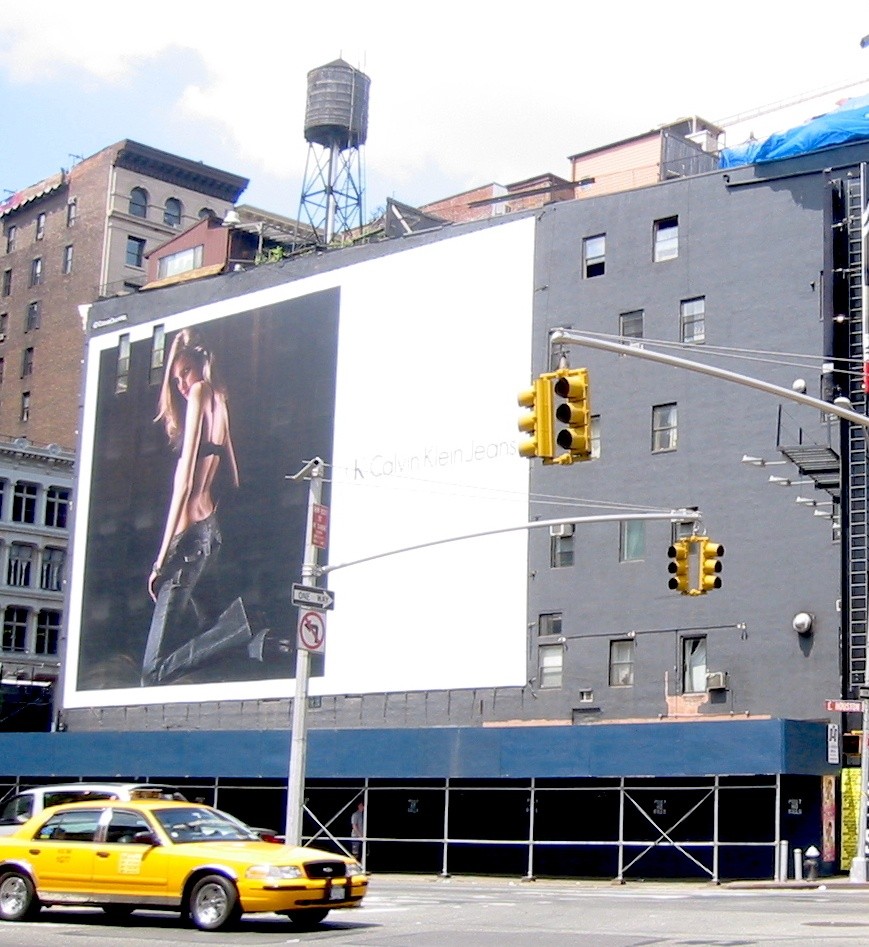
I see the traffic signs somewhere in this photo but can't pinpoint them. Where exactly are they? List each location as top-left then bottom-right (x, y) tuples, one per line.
(292, 582), (335, 611)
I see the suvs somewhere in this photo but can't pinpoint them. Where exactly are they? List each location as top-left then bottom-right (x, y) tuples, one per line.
(0, 782), (201, 833)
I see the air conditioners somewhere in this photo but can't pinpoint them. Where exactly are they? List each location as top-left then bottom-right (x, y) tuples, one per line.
(706, 672), (726, 691)
(550, 524), (573, 538)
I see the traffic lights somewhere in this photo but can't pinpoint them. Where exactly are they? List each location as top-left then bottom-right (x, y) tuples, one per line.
(698, 541), (724, 592)
(553, 372), (591, 456)
(667, 543), (690, 592)
(516, 378), (556, 459)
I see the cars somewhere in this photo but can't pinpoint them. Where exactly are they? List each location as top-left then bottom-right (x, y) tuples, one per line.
(0, 790), (369, 932)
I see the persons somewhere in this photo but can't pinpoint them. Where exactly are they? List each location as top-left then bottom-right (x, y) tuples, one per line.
(351, 802), (372, 875)
(142, 325), (294, 689)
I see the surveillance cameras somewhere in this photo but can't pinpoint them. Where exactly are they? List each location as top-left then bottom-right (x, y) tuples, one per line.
(293, 458), (320, 483)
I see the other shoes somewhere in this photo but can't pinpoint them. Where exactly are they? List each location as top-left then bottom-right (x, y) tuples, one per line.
(263, 633), (295, 664)
